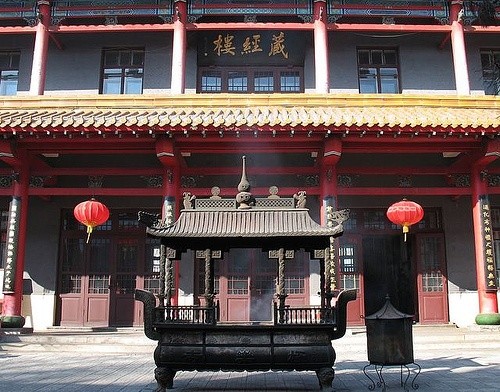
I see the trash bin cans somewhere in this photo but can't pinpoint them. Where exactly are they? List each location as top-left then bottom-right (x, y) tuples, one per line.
(361, 294), (422, 391)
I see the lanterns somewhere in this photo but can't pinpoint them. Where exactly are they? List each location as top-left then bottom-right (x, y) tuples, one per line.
(73, 195), (110, 244)
(386, 196), (424, 241)
